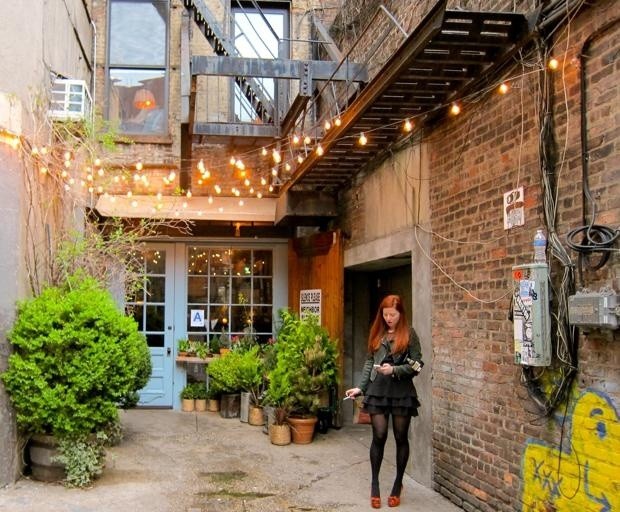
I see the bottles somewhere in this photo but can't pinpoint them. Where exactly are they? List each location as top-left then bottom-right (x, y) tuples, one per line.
(532, 231), (547, 263)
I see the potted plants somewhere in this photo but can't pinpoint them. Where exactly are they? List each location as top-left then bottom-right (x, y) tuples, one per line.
(179, 305), (337, 445)
(4, 269), (152, 482)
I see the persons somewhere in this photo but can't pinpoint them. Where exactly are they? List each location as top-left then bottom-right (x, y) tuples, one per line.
(134, 298), (272, 348)
(345, 294), (425, 509)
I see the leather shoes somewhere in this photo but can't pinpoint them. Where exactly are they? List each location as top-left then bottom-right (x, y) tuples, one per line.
(388, 484), (403, 507)
(371, 496), (381, 508)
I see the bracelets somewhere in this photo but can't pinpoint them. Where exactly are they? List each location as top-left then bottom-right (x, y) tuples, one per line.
(391, 366), (395, 377)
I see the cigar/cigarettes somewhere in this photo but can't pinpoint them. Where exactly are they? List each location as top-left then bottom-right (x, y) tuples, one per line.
(342, 396), (351, 401)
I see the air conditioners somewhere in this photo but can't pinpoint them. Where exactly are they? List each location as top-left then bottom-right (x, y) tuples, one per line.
(47, 76), (94, 139)
